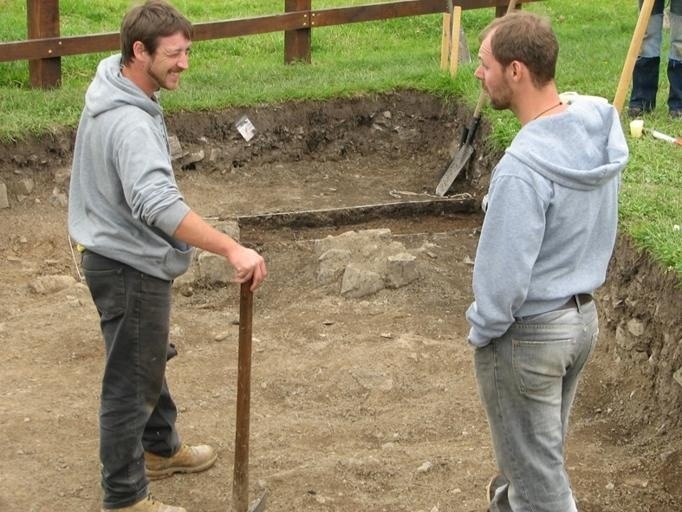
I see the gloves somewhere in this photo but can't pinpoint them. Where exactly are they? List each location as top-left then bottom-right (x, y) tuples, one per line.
(564, 294), (592, 307)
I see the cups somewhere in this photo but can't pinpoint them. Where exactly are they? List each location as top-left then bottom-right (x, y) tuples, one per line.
(630, 119), (643, 138)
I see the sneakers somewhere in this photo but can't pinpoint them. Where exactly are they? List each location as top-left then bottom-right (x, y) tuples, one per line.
(668, 107), (682, 115)
(630, 107), (643, 117)
(487, 474), (507, 504)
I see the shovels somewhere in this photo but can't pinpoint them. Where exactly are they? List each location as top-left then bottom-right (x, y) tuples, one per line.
(435, 0), (517, 197)
(445, 0), (471, 67)
(630, 119), (682, 146)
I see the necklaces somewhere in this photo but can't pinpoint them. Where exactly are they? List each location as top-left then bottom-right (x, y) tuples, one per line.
(532, 101), (563, 120)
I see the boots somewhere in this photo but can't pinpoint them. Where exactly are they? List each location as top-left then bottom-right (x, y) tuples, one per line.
(103, 497), (187, 512)
(144, 444), (217, 481)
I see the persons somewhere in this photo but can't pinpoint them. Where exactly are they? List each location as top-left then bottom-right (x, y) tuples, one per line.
(466, 9), (629, 512)
(628, 0), (682, 117)
(68, 0), (268, 512)
